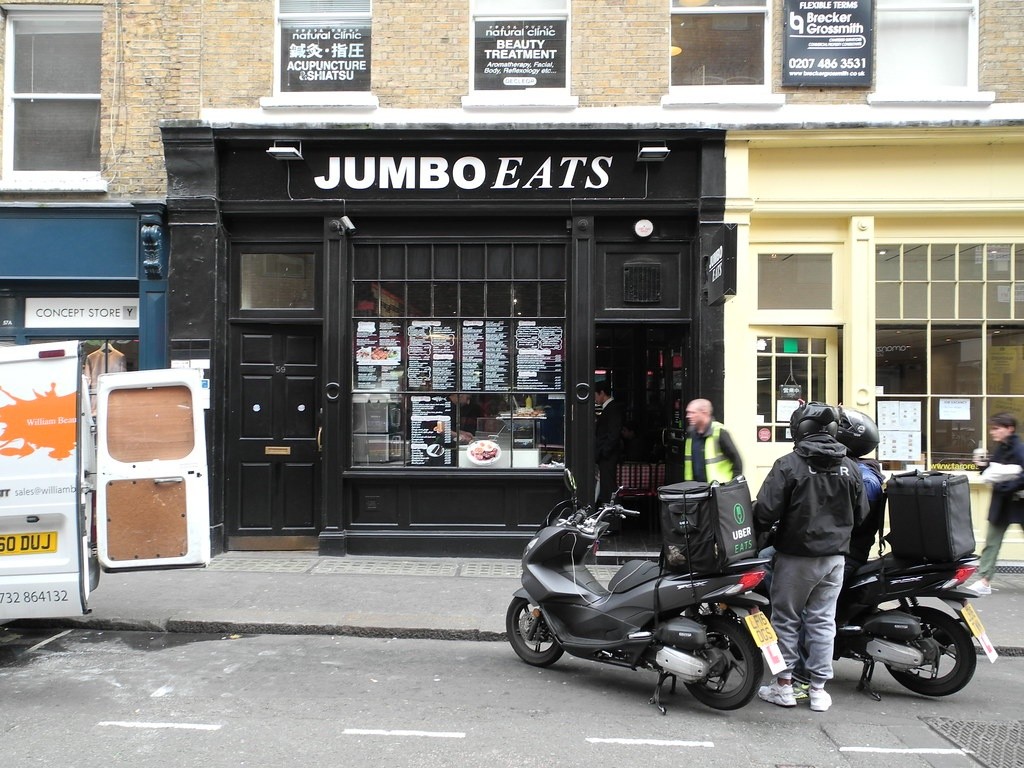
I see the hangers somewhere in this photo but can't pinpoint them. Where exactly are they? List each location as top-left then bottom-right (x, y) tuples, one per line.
(99, 341), (114, 351)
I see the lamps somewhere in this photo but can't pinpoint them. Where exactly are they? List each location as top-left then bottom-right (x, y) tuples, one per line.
(265, 139), (303, 161)
(671, 36), (682, 57)
(636, 140), (671, 162)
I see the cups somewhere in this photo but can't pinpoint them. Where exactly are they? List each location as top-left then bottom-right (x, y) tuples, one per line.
(973, 448), (989, 467)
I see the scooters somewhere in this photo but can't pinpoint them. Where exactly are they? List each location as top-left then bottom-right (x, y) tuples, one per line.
(745, 492), (983, 700)
(504, 455), (772, 715)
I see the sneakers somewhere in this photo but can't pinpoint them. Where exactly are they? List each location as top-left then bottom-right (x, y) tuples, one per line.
(757, 677), (799, 707)
(808, 687), (832, 712)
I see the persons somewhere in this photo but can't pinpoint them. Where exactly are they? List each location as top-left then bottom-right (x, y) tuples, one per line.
(594, 382), (624, 517)
(965, 414), (1024, 595)
(776, 408), (885, 700)
(748, 401), (863, 711)
(621, 420), (648, 462)
(675, 399), (743, 491)
(426, 390), (474, 467)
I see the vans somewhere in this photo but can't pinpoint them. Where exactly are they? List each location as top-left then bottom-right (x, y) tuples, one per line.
(1, 342), (196, 609)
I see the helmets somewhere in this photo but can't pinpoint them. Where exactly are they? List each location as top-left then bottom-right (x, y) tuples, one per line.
(832, 404), (881, 458)
(788, 401), (840, 444)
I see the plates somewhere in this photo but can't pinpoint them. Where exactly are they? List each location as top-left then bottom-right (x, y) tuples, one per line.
(466, 440), (501, 466)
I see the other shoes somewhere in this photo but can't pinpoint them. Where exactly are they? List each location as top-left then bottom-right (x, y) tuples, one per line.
(966, 581), (993, 595)
